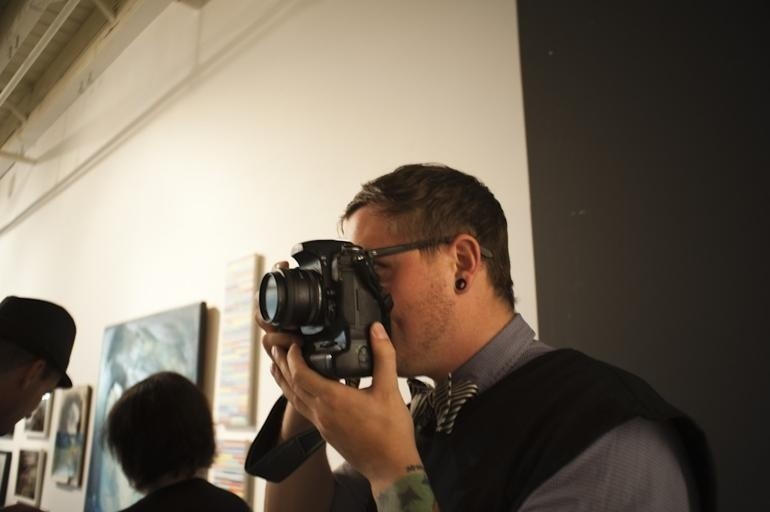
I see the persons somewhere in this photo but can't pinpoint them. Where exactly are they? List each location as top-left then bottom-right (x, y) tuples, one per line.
(0, 296), (76, 437)
(254, 165), (716, 512)
(53, 392), (83, 484)
(106, 371), (252, 512)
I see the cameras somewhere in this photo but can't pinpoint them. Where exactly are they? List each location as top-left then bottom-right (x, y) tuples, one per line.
(258, 239), (393, 379)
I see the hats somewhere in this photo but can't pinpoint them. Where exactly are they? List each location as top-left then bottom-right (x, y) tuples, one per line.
(1, 296), (76, 388)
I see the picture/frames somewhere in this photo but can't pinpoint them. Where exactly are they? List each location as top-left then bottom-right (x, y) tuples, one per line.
(11, 390), (54, 508)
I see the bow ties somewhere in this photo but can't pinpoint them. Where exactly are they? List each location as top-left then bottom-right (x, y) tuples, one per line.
(407, 372), (478, 435)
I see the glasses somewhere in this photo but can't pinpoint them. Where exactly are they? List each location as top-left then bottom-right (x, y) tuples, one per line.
(365, 235), (494, 259)
